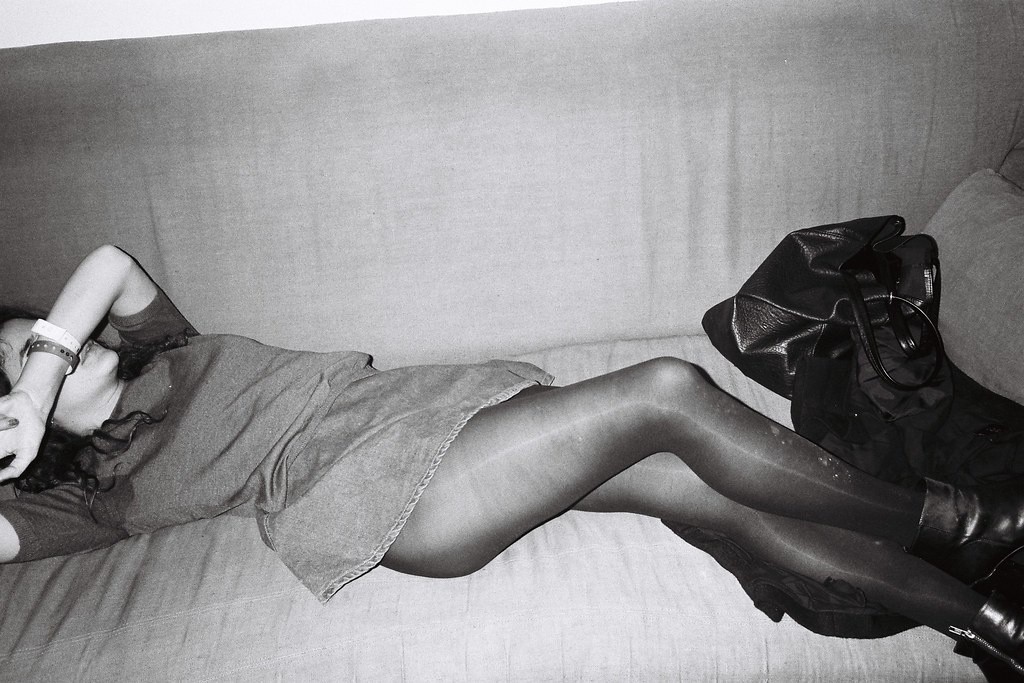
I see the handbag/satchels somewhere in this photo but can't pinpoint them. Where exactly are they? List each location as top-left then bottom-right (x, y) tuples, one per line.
(704, 215), (942, 401)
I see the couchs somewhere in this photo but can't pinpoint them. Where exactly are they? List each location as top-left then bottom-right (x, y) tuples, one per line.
(0, 0), (1024, 683)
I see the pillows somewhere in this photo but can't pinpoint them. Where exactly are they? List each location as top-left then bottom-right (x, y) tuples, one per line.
(921, 167), (1024, 408)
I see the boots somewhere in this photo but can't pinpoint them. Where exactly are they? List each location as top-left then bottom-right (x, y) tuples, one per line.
(952, 588), (1024, 683)
(905, 474), (1023, 594)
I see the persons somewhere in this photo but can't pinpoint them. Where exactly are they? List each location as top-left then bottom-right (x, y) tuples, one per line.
(0, 246), (1024, 683)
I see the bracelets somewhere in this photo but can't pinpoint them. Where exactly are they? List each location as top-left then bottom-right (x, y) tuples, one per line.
(20, 318), (82, 375)
